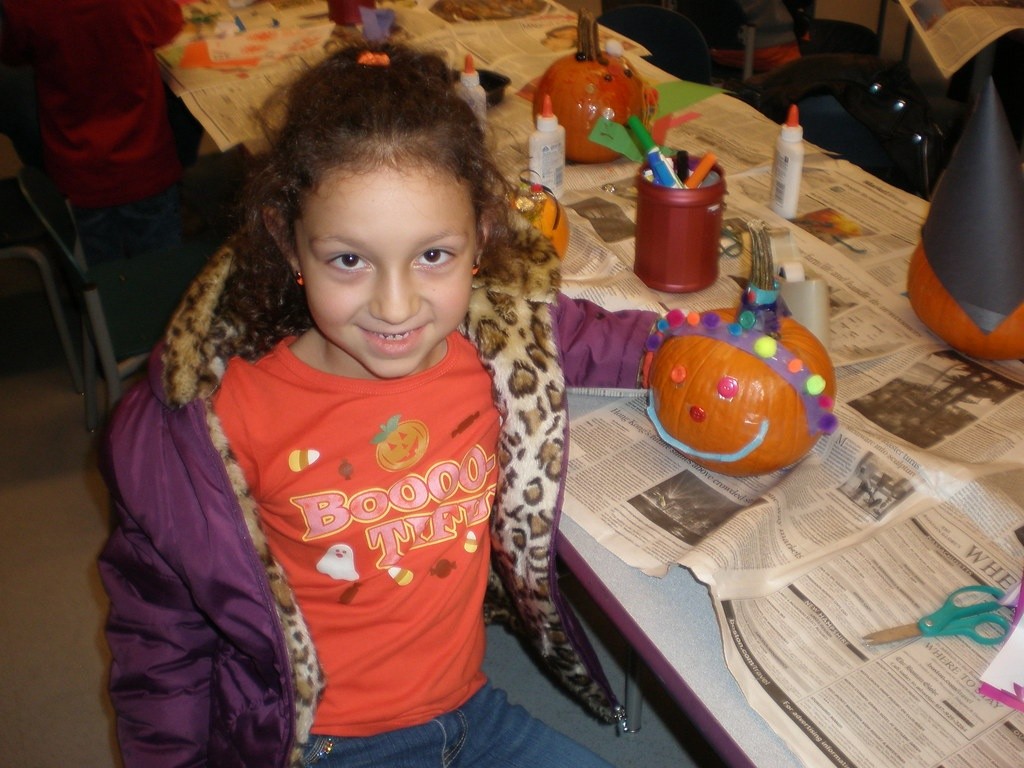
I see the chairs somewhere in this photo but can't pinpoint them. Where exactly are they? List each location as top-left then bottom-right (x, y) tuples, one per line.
(797, 19), (880, 56)
(596, 6), (712, 86)
(17, 161), (218, 432)
(0, 133), (85, 396)
(727, 51), (943, 198)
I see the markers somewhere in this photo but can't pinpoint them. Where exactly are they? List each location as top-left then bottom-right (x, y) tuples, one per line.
(626, 112), (722, 192)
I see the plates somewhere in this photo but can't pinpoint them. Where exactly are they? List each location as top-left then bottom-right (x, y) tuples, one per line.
(453, 68), (512, 108)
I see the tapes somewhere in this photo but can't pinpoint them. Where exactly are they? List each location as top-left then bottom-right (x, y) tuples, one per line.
(776, 261), (806, 281)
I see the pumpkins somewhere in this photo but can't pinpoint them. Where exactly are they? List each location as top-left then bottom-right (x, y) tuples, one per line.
(907, 243), (1024, 361)
(648, 218), (838, 480)
(532, 8), (648, 164)
(507, 188), (570, 262)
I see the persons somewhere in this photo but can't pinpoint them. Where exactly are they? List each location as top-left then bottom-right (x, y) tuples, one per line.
(709, 0), (811, 73)
(0, 0), (203, 378)
(97, 38), (661, 768)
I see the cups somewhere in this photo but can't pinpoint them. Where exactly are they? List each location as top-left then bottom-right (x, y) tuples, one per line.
(328, 0), (374, 25)
(634, 156), (724, 294)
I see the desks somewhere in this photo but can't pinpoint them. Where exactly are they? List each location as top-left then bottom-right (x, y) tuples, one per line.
(144, 0), (1024, 768)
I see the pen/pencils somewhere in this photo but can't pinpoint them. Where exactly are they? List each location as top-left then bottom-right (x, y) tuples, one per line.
(235, 15), (246, 31)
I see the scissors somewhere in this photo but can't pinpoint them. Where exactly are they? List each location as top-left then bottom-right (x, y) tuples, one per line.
(861, 585), (1011, 648)
